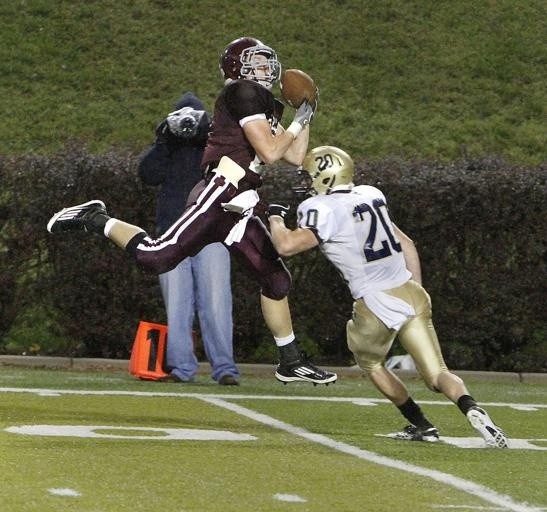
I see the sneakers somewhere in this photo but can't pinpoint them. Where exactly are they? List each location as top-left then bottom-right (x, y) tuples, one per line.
(276, 351), (338, 386)
(385, 425), (439, 441)
(46, 200), (107, 238)
(467, 405), (508, 448)
(159, 375), (179, 381)
(220, 374), (238, 385)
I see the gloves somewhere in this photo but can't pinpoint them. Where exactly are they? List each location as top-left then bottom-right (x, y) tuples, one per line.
(293, 88), (319, 128)
(265, 201), (290, 220)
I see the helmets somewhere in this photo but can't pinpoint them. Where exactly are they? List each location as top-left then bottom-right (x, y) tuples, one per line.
(290, 145), (354, 203)
(219, 37), (275, 90)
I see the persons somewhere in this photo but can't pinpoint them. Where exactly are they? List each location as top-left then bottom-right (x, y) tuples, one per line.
(138, 90), (240, 384)
(264, 146), (510, 449)
(47, 36), (338, 384)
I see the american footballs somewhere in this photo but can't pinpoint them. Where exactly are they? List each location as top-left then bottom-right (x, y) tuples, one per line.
(280, 69), (317, 108)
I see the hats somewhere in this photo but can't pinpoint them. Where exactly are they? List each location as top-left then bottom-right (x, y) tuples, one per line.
(176, 93), (208, 128)
